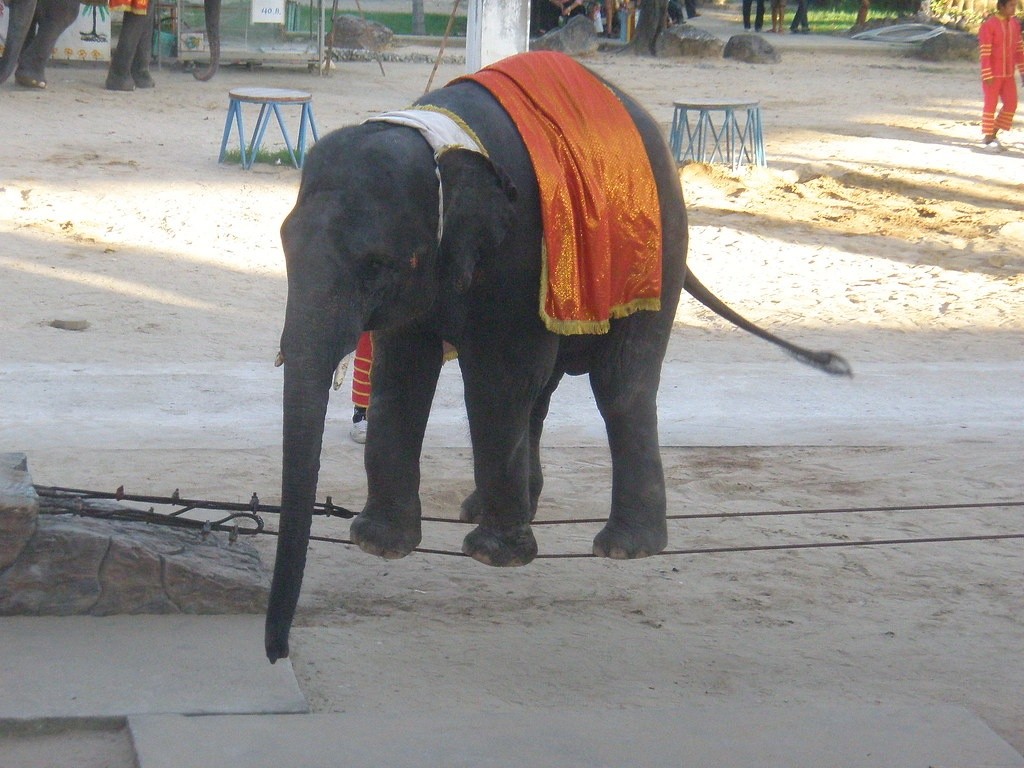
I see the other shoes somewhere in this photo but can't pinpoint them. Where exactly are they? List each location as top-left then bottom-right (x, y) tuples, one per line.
(802, 27), (810, 33)
(790, 27), (802, 33)
(745, 28), (751, 33)
(985, 137), (1007, 154)
(665, 11), (701, 27)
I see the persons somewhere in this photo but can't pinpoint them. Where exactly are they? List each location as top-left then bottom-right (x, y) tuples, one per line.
(536, 0), (613, 37)
(767, 0), (787, 34)
(668, 0), (686, 25)
(976, 0), (1024, 150)
(743, 0), (765, 33)
(790, 0), (811, 34)
(686, 0), (701, 18)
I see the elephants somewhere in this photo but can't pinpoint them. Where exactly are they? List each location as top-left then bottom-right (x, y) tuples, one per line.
(264, 49), (855, 666)
(0, 0), (222, 92)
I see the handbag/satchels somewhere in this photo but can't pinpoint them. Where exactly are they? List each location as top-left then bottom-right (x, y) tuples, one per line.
(558, 14), (567, 27)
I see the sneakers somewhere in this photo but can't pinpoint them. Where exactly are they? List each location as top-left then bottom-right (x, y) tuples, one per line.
(350, 416), (368, 443)
